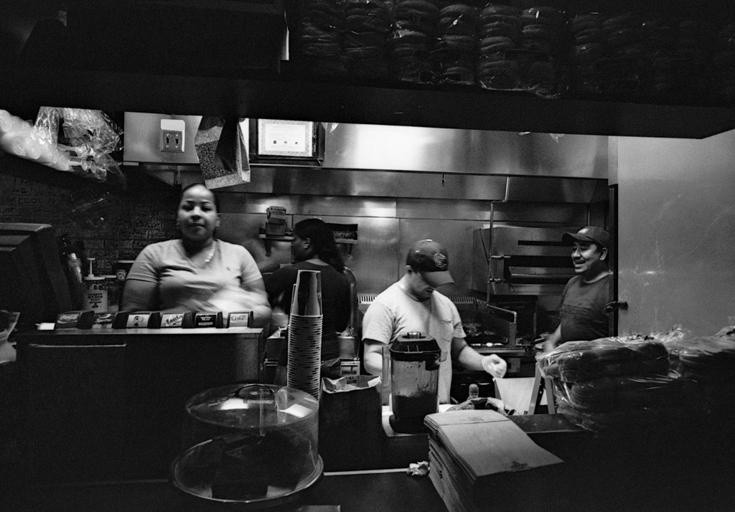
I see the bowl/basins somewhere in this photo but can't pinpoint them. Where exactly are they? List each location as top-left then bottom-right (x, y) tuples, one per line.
(169, 438), (324, 504)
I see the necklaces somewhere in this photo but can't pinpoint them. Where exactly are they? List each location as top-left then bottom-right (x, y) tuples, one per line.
(201, 241), (218, 268)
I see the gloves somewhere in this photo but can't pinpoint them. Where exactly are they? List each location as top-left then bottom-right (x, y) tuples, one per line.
(481, 354), (507, 384)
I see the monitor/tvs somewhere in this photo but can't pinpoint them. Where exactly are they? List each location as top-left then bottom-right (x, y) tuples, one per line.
(0, 222), (72, 327)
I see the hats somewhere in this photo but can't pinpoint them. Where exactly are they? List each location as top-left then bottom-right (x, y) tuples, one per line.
(561, 225), (611, 251)
(407, 239), (456, 288)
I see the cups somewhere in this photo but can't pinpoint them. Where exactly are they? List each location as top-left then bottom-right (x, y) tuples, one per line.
(288, 268), (324, 404)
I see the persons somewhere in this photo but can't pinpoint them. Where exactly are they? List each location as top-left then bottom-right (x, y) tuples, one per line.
(265, 217), (351, 386)
(121, 181), (271, 319)
(542, 224), (615, 353)
(361, 238), (509, 404)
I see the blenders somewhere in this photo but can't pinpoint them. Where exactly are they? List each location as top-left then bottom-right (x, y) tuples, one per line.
(386, 332), (444, 435)
